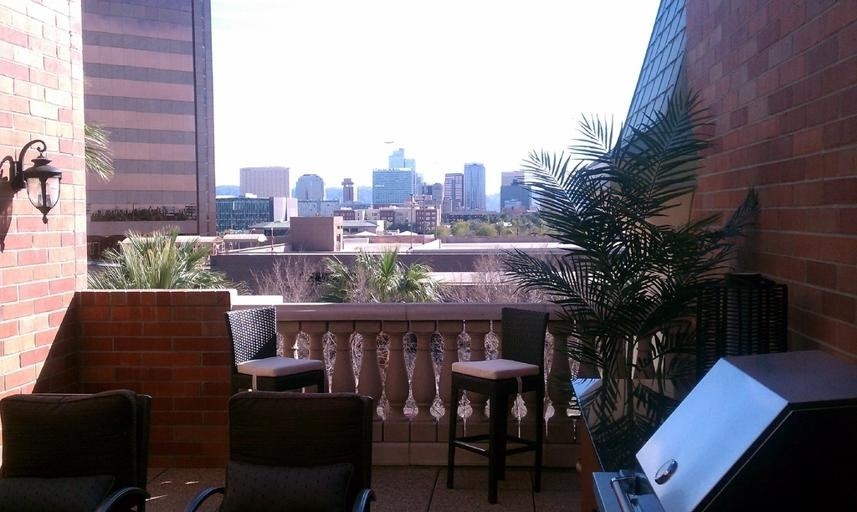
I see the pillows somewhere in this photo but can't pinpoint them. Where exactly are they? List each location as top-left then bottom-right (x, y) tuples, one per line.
(0, 389), (137, 495)
(229, 392), (365, 468)
(0, 475), (117, 512)
(219, 461), (353, 511)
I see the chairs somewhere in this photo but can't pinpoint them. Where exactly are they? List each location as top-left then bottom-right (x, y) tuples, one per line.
(446, 307), (549, 505)
(0, 389), (153, 512)
(223, 305), (325, 394)
(185, 391), (377, 512)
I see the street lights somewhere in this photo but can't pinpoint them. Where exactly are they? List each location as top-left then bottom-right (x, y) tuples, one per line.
(422, 193), (425, 245)
(408, 193), (413, 248)
(433, 199), (437, 240)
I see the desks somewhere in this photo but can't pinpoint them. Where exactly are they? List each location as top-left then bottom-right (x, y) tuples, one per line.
(571, 376), (700, 512)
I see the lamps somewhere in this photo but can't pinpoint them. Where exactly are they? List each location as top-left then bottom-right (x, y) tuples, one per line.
(9, 139), (63, 224)
